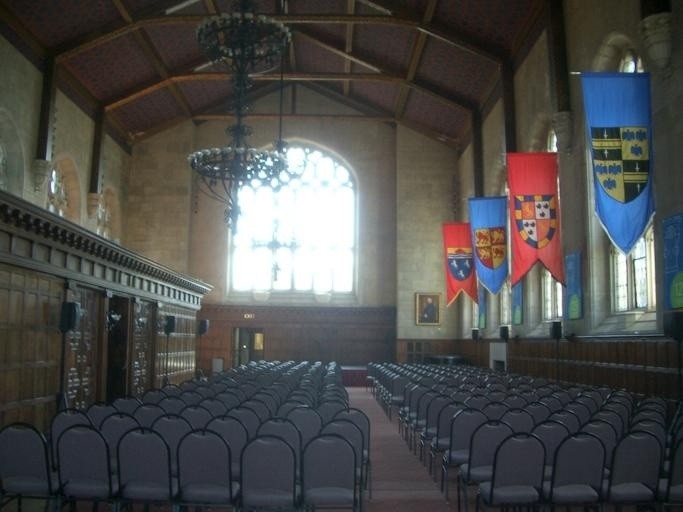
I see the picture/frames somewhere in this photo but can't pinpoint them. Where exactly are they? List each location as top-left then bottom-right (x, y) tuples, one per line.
(415, 292), (442, 326)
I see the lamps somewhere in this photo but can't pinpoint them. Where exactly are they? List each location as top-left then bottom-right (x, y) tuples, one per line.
(178, 6), (299, 235)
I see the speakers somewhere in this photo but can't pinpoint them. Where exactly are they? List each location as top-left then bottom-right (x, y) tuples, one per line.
(663, 310), (683, 340)
(472, 330), (479, 341)
(199, 319), (209, 335)
(63, 302), (81, 330)
(499, 325), (509, 341)
(552, 321), (561, 338)
(164, 316), (176, 334)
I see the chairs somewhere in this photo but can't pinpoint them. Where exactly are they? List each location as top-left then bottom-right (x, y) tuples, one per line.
(367, 361), (683, 511)
(1, 360), (370, 511)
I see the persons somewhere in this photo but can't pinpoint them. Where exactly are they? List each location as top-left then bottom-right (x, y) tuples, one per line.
(422, 296), (435, 323)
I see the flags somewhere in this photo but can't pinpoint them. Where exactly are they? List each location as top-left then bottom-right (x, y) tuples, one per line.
(443, 224), (479, 307)
(511, 153), (566, 289)
(469, 197), (509, 295)
(581, 73), (658, 254)
